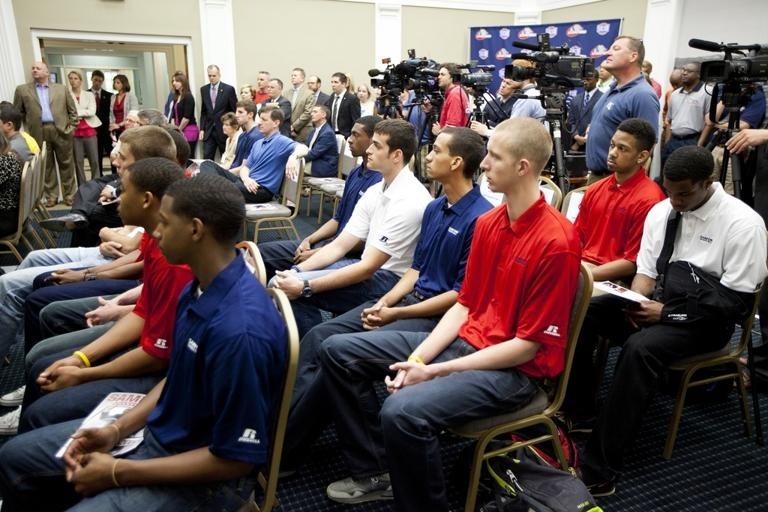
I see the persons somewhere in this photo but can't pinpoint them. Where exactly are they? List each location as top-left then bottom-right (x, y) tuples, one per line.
(398, 59), (547, 199)
(320, 115), (582, 511)
(270, 119), (434, 340)
(199, 65), (239, 161)
(110, 75), (139, 141)
(662, 61), (768, 222)
(1, 102), (190, 434)
(585, 33), (661, 185)
(641, 61), (662, 99)
(572, 119), (665, 282)
(281, 125), (487, 472)
(562, 146), (767, 496)
(222, 68), (377, 204)
(3, 173), (289, 511)
(13, 61), (80, 206)
(88, 69), (117, 175)
(68, 71), (101, 186)
(164, 72), (200, 168)
(19, 158), (194, 437)
(564, 60), (617, 190)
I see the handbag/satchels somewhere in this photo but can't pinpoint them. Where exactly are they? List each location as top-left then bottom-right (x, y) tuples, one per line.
(656, 259), (757, 325)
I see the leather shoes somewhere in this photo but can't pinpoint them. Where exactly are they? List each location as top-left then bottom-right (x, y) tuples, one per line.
(38, 214), (89, 233)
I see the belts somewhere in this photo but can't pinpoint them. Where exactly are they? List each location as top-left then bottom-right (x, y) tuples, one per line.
(42, 121), (54, 126)
(413, 290), (425, 301)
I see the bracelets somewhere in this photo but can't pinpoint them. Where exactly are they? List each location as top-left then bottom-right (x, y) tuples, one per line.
(112, 460), (121, 485)
(73, 351), (90, 366)
(83, 273), (87, 281)
(110, 424), (120, 442)
(291, 267), (299, 272)
(88, 272), (96, 281)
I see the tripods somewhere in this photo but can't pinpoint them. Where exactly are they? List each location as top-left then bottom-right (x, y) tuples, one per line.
(414, 98), (444, 157)
(407, 93), (429, 183)
(704, 105), (763, 448)
(532, 120), (567, 199)
(382, 96), (404, 120)
(434, 109), (492, 198)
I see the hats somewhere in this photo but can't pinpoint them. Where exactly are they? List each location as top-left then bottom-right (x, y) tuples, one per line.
(512, 59), (534, 84)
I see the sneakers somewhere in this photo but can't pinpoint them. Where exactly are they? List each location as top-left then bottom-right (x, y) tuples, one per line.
(553, 410), (592, 434)
(568, 467), (616, 497)
(0, 383), (27, 407)
(0, 407), (21, 434)
(327, 469), (394, 505)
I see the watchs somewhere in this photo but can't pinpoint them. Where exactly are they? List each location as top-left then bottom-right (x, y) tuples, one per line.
(301, 280), (313, 298)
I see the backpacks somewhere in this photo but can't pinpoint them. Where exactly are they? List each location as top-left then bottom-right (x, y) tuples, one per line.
(480, 450), (603, 512)
(485, 408), (578, 471)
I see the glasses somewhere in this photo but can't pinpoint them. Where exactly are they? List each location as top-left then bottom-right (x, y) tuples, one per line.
(637, 39), (642, 52)
(683, 69), (696, 73)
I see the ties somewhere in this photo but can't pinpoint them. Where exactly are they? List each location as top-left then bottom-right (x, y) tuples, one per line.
(212, 87), (216, 109)
(332, 96), (339, 131)
(584, 95), (589, 106)
(95, 92), (100, 112)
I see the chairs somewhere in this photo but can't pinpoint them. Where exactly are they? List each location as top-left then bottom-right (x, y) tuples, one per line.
(439, 258), (594, 510)
(241, 153), (306, 244)
(592, 227), (761, 460)
(299, 134), (415, 224)
(0, 141), (59, 268)
(225, 241), (300, 508)
(477, 169), (587, 225)
